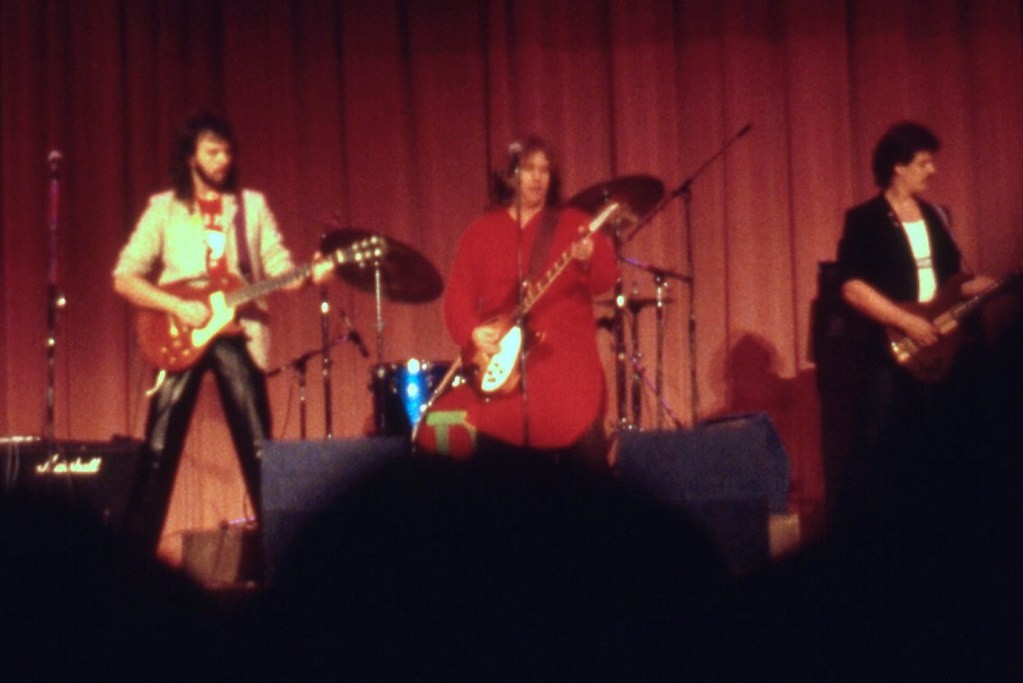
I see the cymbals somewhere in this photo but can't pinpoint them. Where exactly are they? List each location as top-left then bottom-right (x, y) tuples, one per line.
(597, 293), (676, 306)
(321, 227), (445, 304)
(568, 174), (665, 231)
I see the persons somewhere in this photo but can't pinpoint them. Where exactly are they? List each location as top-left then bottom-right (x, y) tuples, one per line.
(109, 109), (335, 593)
(829, 121), (1001, 510)
(446, 135), (656, 604)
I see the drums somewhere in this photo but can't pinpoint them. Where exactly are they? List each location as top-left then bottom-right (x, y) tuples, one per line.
(367, 357), (455, 435)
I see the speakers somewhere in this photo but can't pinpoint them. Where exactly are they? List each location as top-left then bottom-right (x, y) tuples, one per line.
(610, 414), (790, 515)
(0, 438), (143, 526)
(259, 438), (406, 566)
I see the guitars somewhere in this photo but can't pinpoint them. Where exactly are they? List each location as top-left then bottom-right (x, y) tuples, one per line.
(459, 195), (631, 403)
(884, 268), (1023, 384)
(134, 234), (389, 373)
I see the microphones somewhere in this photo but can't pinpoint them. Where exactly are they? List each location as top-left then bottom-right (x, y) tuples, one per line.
(341, 312), (370, 358)
(47, 150), (62, 173)
(508, 142), (523, 173)
